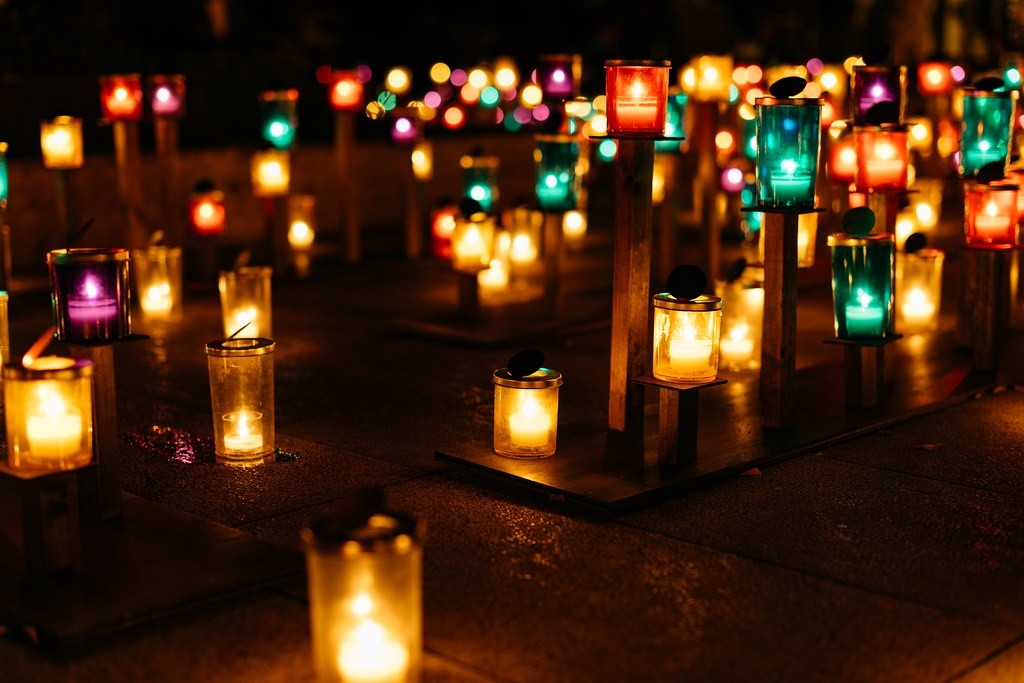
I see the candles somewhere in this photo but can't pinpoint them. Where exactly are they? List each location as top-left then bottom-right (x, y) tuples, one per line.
(508, 399), (552, 446)
(335, 621), (410, 681)
(25, 392), (82, 458)
(720, 322), (753, 363)
(225, 408), (263, 451)
(671, 328), (712, 372)
(1, 57), (1021, 335)
